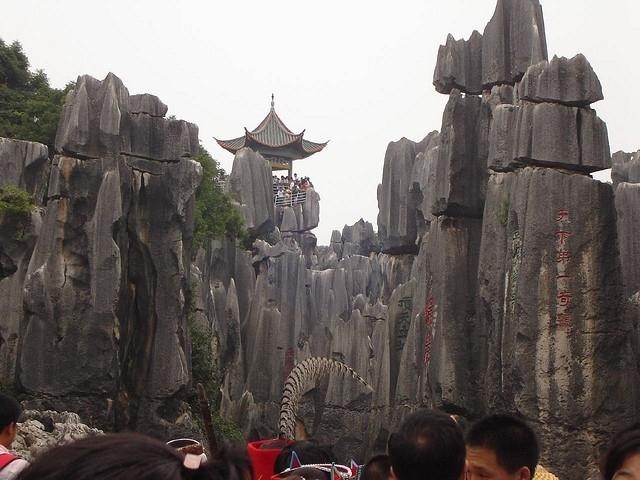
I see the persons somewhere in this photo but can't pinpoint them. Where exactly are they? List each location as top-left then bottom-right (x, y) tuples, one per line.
(273, 173), (313, 208)
